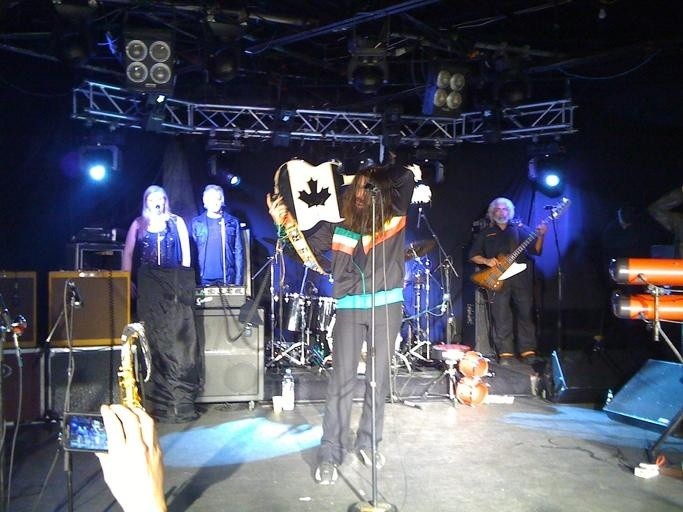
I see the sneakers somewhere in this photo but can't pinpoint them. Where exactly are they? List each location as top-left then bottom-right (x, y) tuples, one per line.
(313, 461), (338, 486)
(360, 446), (385, 469)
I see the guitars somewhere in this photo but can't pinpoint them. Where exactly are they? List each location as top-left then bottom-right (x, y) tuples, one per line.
(469, 197), (570, 294)
(272, 155), (423, 238)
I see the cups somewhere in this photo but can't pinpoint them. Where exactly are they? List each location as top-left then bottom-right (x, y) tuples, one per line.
(272, 395), (284, 413)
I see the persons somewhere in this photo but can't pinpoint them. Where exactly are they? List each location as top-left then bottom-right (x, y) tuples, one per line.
(188, 183), (245, 288)
(467, 196), (546, 364)
(89, 402), (168, 512)
(74, 419), (102, 448)
(263, 162), (416, 486)
(119, 184), (191, 300)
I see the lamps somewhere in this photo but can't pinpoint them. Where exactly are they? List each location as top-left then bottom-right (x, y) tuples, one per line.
(420, 60), (473, 120)
(343, 20), (392, 97)
(121, 28), (178, 95)
(195, 9), (253, 85)
(210, 148), (242, 187)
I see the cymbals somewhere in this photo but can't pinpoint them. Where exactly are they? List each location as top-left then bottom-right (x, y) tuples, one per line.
(405, 240), (436, 262)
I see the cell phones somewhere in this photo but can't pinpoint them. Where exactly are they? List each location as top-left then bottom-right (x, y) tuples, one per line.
(62, 411), (109, 453)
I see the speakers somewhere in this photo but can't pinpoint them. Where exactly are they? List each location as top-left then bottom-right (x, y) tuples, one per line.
(192, 21), (242, 83)
(422, 61), (472, 118)
(348, 47), (390, 94)
(122, 27), (175, 93)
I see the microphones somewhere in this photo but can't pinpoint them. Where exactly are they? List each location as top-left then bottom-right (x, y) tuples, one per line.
(68, 279), (84, 311)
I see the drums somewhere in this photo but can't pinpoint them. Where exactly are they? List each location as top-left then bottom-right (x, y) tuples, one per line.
(327, 311), (402, 375)
(276, 293), (338, 332)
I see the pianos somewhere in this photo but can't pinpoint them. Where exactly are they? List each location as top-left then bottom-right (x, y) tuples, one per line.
(67, 226), (127, 245)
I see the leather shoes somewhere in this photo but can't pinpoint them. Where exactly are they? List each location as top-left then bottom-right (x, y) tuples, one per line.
(498, 350), (535, 359)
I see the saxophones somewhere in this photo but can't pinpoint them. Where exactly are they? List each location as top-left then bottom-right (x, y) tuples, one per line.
(118, 321), (147, 410)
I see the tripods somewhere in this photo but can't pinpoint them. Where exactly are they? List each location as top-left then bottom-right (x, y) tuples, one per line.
(388, 269), (464, 410)
(265, 264), (331, 379)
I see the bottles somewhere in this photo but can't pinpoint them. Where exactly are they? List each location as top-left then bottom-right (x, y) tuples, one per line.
(282, 369), (295, 412)
